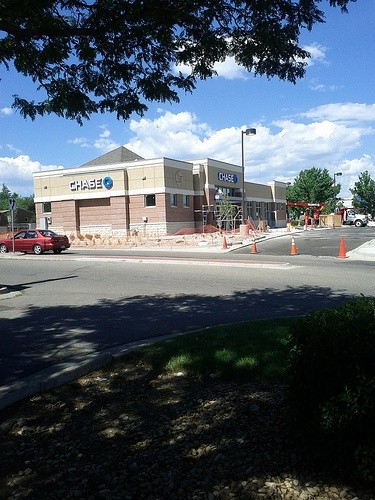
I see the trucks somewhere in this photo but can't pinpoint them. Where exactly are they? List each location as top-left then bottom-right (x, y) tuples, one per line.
(337, 207), (368, 227)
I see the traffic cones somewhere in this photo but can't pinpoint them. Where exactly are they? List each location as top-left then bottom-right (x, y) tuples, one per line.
(222, 237), (228, 249)
(337, 235), (348, 258)
(251, 236), (258, 254)
(290, 237), (298, 254)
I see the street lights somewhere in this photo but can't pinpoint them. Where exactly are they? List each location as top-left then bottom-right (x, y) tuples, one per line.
(239, 128), (257, 235)
(334, 173), (342, 186)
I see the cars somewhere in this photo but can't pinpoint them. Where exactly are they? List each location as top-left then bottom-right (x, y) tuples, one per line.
(0, 229), (70, 255)
